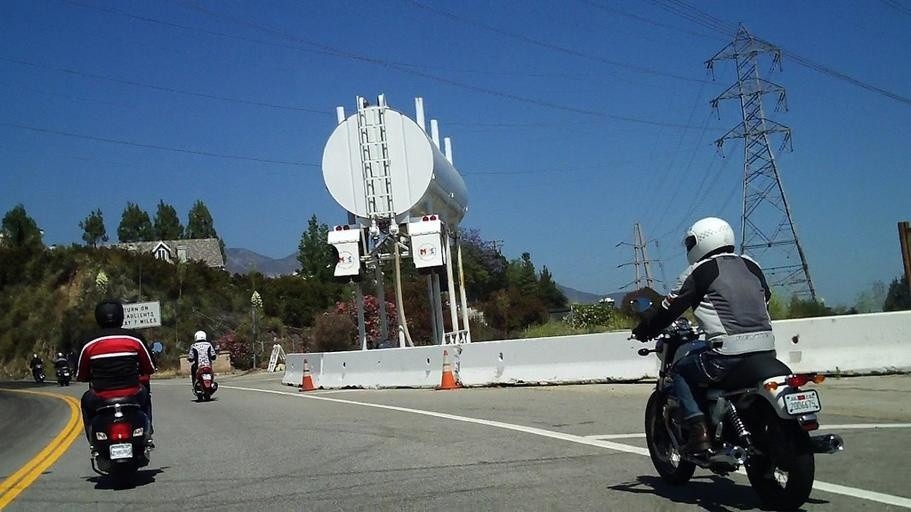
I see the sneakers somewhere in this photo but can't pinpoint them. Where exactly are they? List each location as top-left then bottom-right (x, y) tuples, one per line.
(677, 426), (714, 456)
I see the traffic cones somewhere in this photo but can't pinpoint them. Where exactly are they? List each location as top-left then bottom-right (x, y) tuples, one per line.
(436, 348), (461, 390)
(297, 358), (319, 391)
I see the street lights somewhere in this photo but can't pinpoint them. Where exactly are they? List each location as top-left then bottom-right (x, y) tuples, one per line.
(127, 246), (143, 302)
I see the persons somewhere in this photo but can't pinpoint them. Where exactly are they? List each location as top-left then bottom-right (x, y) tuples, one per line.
(54, 351), (69, 381)
(29, 352), (45, 380)
(75, 299), (158, 456)
(631, 217), (777, 456)
(187, 330), (217, 387)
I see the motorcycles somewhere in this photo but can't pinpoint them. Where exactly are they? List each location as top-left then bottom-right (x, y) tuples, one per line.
(50, 355), (75, 388)
(27, 359), (50, 386)
(81, 340), (167, 476)
(185, 354), (220, 404)
(621, 287), (845, 512)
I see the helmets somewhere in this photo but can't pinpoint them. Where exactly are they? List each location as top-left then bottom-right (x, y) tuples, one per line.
(94, 298), (125, 329)
(193, 329), (208, 343)
(683, 216), (736, 268)
(56, 352), (64, 359)
(33, 353), (38, 359)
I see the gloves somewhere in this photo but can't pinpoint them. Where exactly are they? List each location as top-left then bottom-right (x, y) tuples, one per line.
(631, 325), (654, 343)
